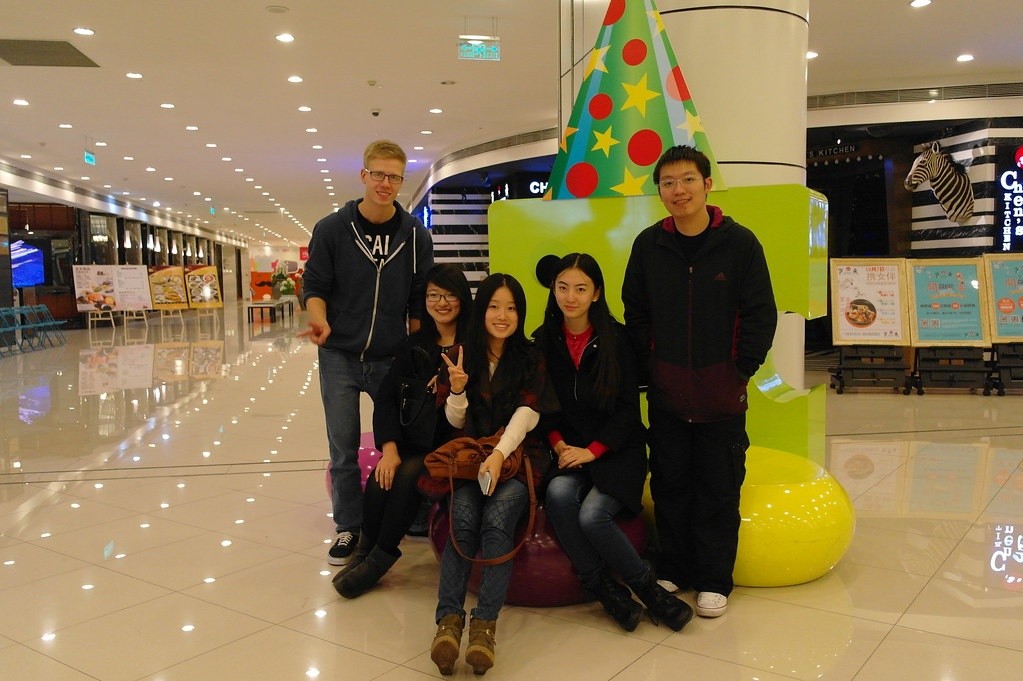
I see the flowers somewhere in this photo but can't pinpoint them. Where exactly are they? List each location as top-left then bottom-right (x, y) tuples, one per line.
(295, 268), (305, 284)
(269, 262), (296, 288)
(280, 278), (296, 295)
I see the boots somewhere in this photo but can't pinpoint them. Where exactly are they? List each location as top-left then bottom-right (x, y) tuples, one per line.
(584, 571), (642, 631)
(465, 619), (497, 675)
(628, 572), (694, 632)
(431, 614), (463, 675)
(332, 527), (403, 599)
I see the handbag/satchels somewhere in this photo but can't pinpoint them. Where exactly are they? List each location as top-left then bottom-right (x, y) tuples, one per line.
(423, 425), (536, 565)
(394, 345), (438, 450)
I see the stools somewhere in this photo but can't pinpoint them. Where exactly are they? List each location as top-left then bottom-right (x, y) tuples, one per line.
(325, 433), (857, 606)
(247, 298), (294, 324)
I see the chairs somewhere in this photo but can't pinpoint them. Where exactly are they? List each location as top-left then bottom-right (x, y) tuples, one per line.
(0, 304), (68, 358)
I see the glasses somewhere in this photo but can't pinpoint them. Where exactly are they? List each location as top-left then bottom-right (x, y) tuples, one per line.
(365, 169), (404, 184)
(659, 175), (703, 189)
(426, 293), (459, 302)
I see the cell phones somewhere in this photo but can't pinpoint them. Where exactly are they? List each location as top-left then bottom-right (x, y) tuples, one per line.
(478, 462), (491, 495)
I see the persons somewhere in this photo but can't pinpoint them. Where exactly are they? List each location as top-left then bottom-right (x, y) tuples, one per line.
(295, 138), (434, 566)
(330, 265), (473, 599)
(539, 253), (695, 633)
(619, 146), (778, 619)
(429, 272), (543, 675)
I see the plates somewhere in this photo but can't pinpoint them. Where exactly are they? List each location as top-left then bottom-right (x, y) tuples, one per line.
(845, 299), (877, 328)
(188, 274), (218, 302)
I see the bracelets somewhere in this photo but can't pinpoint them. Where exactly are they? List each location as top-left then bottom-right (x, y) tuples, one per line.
(450, 389), (466, 395)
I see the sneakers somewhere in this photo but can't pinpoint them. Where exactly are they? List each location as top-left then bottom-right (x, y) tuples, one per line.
(656, 580), (678, 594)
(696, 592), (728, 617)
(328, 531), (360, 565)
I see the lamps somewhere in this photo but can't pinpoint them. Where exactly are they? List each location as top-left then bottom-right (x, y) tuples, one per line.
(92, 218), (204, 259)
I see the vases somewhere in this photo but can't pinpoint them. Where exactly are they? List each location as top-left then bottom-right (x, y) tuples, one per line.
(298, 285), (312, 311)
(271, 279), (292, 311)
(283, 289), (295, 296)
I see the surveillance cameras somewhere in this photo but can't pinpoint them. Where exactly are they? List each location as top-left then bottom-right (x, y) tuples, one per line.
(371, 109), (380, 117)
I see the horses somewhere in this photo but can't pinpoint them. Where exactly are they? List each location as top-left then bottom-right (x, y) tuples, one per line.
(905, 142), (974, 223)
